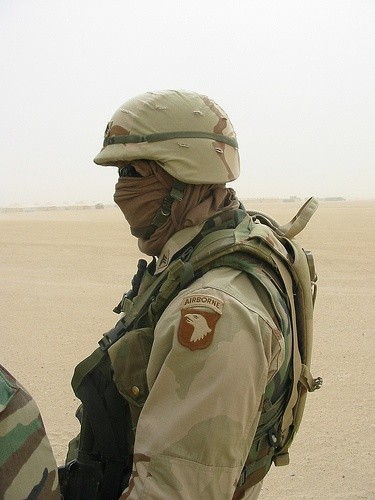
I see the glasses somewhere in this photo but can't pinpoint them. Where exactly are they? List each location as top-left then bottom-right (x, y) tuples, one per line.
(117, 163), (148, 180)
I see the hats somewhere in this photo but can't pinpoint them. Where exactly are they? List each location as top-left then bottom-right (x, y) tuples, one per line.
(92, 91), (239, 184)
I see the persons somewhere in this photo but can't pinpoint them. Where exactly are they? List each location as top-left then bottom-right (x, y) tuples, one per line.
(65, 87), (317, 499)
